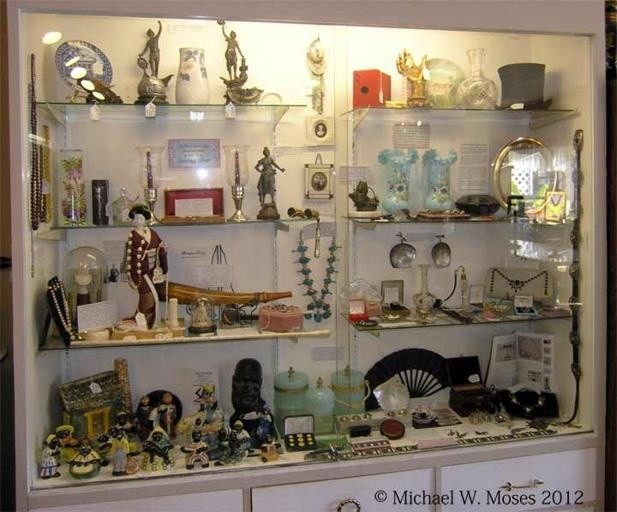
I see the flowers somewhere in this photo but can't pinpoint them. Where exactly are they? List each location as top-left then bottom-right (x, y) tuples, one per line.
(59, 156), (87, 226)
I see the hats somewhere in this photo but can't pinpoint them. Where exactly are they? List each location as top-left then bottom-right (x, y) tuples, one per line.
(494, 63), (552, 110)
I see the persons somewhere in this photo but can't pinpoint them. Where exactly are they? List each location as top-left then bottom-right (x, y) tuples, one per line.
(124, 204), (168, 330)
(254, 148), (285, 205)
(138, 21), (161, 78)
(221, 22), (245, 81)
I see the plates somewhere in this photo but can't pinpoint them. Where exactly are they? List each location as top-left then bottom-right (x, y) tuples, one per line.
(417, 58), (466, 108)
(54, 39), (113, 93)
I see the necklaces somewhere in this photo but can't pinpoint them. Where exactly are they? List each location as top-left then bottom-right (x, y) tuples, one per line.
(30, 54), (39, 230)
(488, 266), (548, 296)
(43, 126), (51, 223)
(509, 392), (546, 412)
(291, 229), (341, 323)
(49, 280), (75, 341)
(39, 145), (46, 222)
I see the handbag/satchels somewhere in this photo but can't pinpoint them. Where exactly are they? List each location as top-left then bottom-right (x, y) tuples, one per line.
(483, 267), (553, 303)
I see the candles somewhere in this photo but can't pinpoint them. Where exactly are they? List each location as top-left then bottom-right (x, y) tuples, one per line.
(146, 151), (153, 187)
(235, 148), (240, 186)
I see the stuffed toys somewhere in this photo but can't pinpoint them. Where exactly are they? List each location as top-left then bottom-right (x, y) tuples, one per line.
(217, 429), (248, 465)
(206, 425), (232, 460)
(192, 300), (212, 324)
(232, 419), (250, 451)
(105, 429), (129, 476)
(180, 430), (208, 469)
(228, 358), (281, 452)
(39, 433), (60, 479)
(157, 391), (177, 435)
(177, 383), (224, 433)
(141, 426), (175, 473)
(138, 395), (154, 439)
(55, 425), (79, 465)
(69, 437), (102, 479)
(108, 410), (139, 456)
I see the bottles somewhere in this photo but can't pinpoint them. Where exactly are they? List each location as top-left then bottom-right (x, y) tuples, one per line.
(91, 178), (110, 225)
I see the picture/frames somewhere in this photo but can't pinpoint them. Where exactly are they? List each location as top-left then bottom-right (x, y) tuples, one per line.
(305, 163), (334, 199)
(306, 114), (334, 145)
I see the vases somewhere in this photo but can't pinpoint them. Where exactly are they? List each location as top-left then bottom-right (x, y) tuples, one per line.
(379, 149), (418, 212)
(412, 265), (436, 312)
(423, 149), (457, 211)
(457, 48), (498, 109)
(174, 44), (209, 106)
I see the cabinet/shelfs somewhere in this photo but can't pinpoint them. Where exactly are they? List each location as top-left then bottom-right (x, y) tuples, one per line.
(26, 486), (245, 512)
(8, 0), (606, 488)
(251, 467), (436, 512)
(439, 446), (598, 512)
